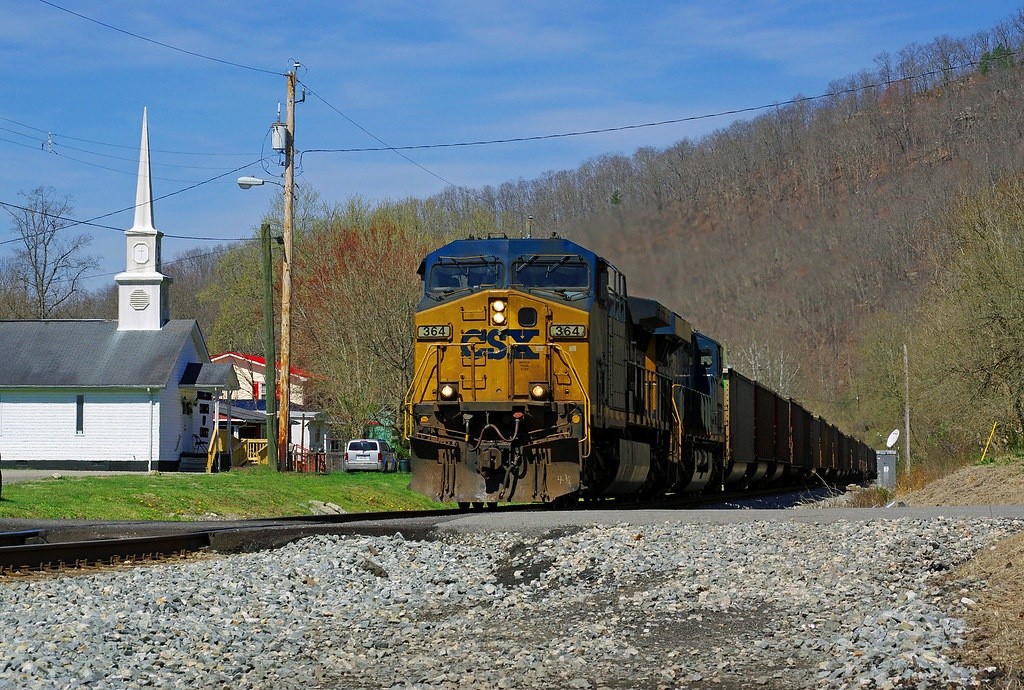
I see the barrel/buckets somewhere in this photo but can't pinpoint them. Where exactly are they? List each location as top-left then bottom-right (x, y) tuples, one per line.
(399, 460), (410, 473)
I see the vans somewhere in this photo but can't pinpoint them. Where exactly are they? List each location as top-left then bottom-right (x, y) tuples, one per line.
(343, 439), (399, 473)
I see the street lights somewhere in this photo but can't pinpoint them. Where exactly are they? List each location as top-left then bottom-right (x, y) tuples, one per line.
(237, 176), (294, 473)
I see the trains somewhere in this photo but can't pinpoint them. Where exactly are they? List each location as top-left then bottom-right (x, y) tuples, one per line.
(402, 235), (878, 510)
